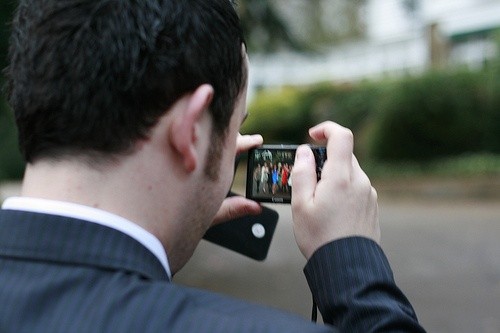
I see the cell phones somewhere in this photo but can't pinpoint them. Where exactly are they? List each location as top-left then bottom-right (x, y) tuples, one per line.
(202, 191), (279, 262)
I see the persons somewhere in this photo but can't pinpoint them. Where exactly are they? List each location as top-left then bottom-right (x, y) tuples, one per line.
(0, 1), (426, 332)
(253, 156), (294, 197)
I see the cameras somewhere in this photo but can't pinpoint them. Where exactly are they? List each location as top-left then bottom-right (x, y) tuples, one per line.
(245, 145), (327, 205)
